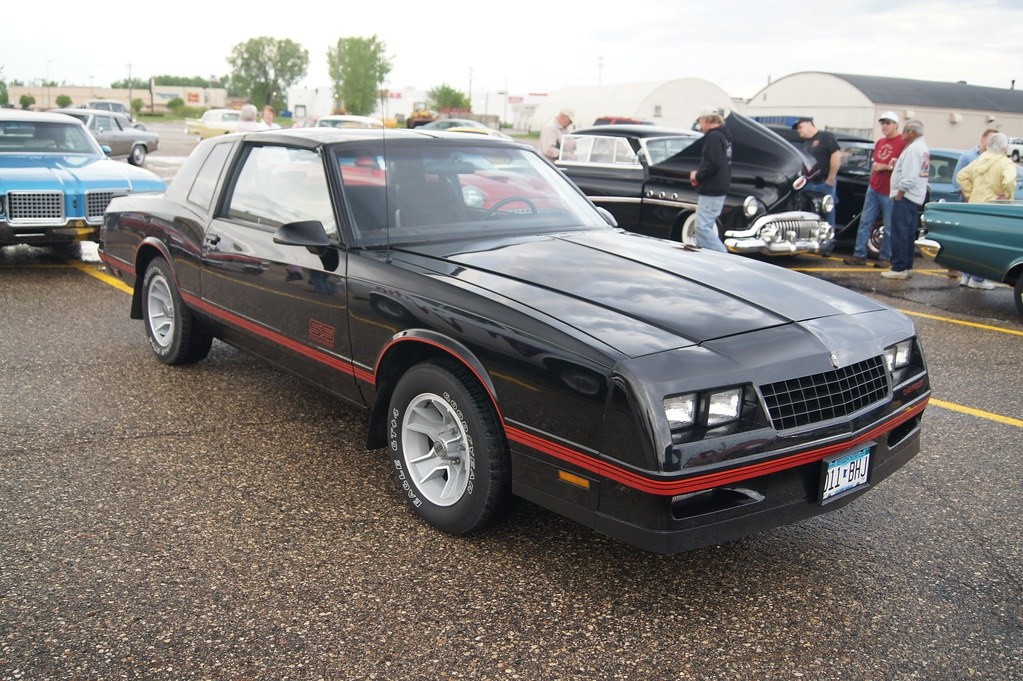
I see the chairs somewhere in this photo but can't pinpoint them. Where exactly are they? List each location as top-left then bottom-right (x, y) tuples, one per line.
(935, 164), (949, 180)
(928, 164), (936, 180)
(396, 178), (468, 227)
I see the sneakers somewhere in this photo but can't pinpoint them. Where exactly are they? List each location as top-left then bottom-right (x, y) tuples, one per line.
(881, 270), (908, 279)
(960, 276), (969, 285)
(907, 270), (912, 277)
(967, 278), (994, 289)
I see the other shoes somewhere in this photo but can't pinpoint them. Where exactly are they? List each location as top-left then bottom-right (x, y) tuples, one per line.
(844, 257), (866, 265)
(874, 258), (890, 268)
(948, 270), (958, 278)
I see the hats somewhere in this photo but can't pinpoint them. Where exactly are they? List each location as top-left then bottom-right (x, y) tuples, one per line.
(792, 118), (812, 130)
(879, 111), (899, 124)
(562, 105), (581, 124)
(696, 107), (718, 122)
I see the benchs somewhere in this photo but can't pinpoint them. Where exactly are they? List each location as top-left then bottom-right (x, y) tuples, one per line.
(0, 138), (57, 149)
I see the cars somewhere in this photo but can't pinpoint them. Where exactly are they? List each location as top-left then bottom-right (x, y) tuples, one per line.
(84, 98), (132, 122)
(0, 106), (166, 257)
(183, 108), (267, 143)
(289, 110), (1023, 322)
(94, 128), (933, 555)
(32, 107), (160, 168)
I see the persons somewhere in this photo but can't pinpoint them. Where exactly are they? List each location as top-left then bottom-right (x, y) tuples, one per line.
(947, 128), (999, 278)
(259, 105), (281, 131)
(880, 121), (929, 279)
(233, 104), (260, 133)
(792, 118), (841, 257)
(305, 268), (339, 297)
(844, 111), (908, 268)
(691, 104), (734, 255)
(537, 107), (577, 164)
(956, 133), (1017, 289)
